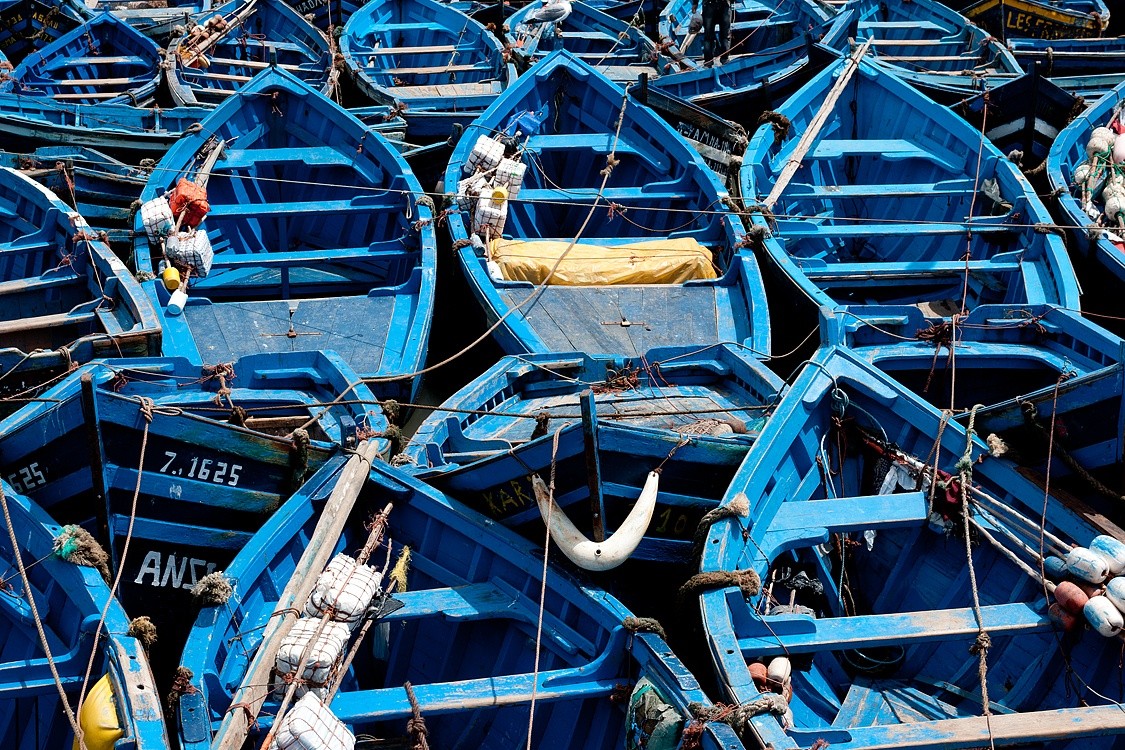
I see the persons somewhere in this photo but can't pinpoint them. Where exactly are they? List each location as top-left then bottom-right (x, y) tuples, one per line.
(692, 0), (733, 68)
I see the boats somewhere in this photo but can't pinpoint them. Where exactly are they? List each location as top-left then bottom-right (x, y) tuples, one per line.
(135, 45), (436, 429)
(1045, 82), (1125, 281)
(174, 449), (747, 750)
(1, 480), (169, 749)
(1, 143), (152, 505)
(439, 31), (772, 365)
(90, 346), (392, 598)
(692, 305), (1125, 750)
(737, 34), (1084, 322)
(392, 341), (796, 564)
(0, 0), (1125, 141)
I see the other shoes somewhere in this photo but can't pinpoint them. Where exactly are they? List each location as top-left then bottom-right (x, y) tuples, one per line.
(704, 61), (713, 69)
(721, 58), (729, 65)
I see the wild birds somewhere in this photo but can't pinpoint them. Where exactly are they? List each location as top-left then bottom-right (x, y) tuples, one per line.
(522, 0), (572, 38)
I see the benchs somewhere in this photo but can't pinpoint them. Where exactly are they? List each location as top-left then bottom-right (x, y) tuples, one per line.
(0, 0), (1125, 750)
